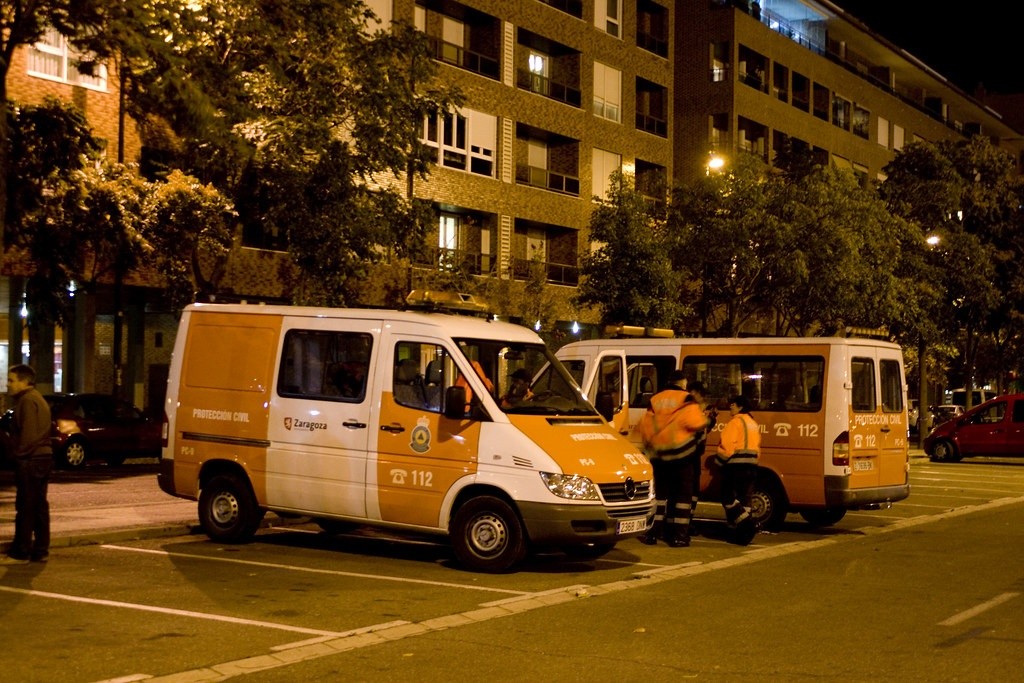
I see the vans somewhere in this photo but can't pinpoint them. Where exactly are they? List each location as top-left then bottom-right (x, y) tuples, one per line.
(153, 288), (658, 573)
(528, 323), (914, 534)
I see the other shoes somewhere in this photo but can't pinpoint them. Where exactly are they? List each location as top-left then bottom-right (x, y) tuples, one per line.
(735, 526), (757, 546)
(638, 534), (656, 545)
(7, 546), (49, 561)
(674, 540), (689, 547)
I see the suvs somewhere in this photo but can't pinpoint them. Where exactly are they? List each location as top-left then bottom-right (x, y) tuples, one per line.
(907, 398), (937, 434)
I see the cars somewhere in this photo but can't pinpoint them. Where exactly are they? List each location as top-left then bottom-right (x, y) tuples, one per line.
(923, 393), (1024, 464)
(0, 392), (163, 470)
(936, 404), (964, 418)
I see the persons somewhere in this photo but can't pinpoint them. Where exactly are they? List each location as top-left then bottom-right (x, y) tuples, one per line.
(6, 364), (53, 563)
(457, 357), (497, 415)
(499, 367), (536, 408)
(641, 368), (762, 548)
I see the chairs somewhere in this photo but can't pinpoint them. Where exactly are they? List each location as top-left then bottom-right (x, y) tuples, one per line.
(630, 376), (655, 408)
(393, 357), (425, 408)
(424, 361), (441, 409)
(455, 360), (495, 411)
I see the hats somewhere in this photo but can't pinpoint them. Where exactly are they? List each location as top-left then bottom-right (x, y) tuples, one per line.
(506, 369), (532, 382)
(669, 370), (686, 381)
(687, 380), (712, 395)
